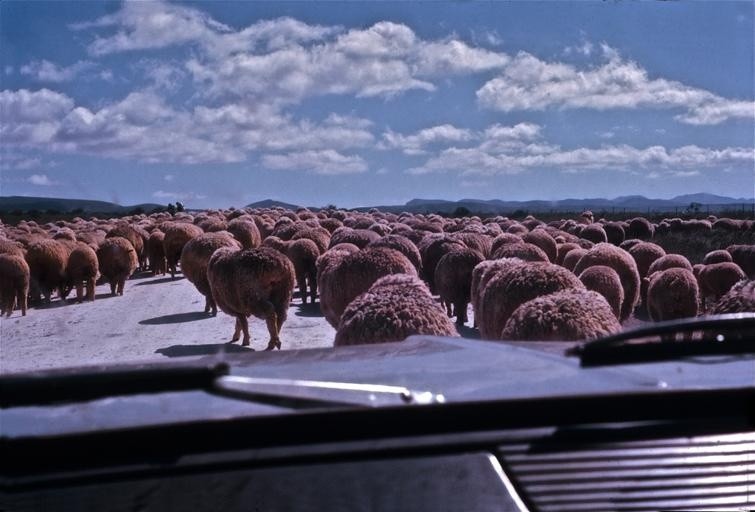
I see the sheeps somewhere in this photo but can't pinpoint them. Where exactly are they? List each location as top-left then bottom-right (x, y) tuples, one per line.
(0, 203), (755, 349)
